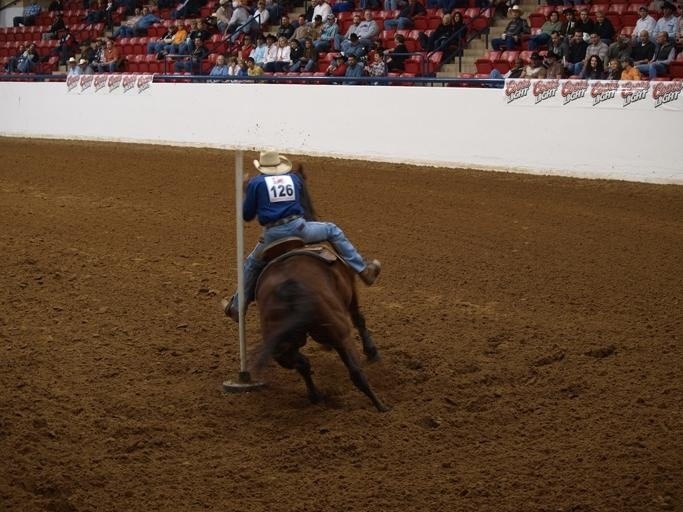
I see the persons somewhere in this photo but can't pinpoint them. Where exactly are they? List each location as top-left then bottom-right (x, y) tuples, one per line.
(0, 0), (519, 90)
(489, 1), (683, 91)
(218, 147), (382, 323)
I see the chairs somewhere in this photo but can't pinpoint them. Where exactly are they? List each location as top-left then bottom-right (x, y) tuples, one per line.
(1, 0), (683, 89)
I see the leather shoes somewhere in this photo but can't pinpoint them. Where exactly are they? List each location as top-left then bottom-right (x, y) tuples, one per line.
(361, 260), (380, 285)
(222, 299), (239, 322)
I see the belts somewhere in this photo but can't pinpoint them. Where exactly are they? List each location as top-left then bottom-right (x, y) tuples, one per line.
(266, 215), (299, 228)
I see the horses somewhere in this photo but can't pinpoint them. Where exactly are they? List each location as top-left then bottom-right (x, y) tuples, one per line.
(250, 160), (393, 414)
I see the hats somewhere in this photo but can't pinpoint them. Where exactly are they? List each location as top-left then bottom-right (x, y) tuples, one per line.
(530, 50), (558, 60)
(253, 147), (292, 174)
(66, 57), (87, 65)
(348, 33), (360, 40)
(508, 5), (524, 15)
(617, 35), (630, 43)
(562, 8), (577, 14)
(315, 14), (335, 20)
(661, 2), (676, 10)
